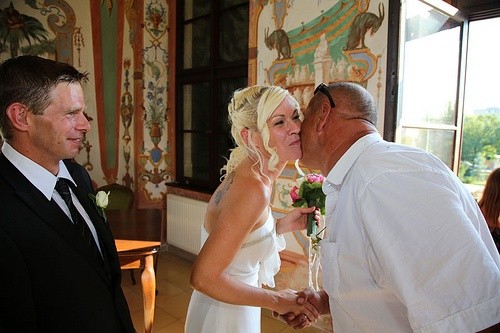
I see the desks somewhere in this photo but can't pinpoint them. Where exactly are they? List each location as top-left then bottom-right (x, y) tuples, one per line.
(96, 240), (162, 333)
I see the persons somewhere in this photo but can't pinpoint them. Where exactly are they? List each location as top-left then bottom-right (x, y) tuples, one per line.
(183, 85), (321, 333)
(0, 55), (136, 333)
(477, 168), (500, 253)
(271, 83), (500, 333)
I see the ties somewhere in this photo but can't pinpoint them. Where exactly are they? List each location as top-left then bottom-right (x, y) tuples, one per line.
(55, 178), (104, 262)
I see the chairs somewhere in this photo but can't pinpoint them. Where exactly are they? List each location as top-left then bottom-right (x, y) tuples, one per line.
(96, 184), (138, 285)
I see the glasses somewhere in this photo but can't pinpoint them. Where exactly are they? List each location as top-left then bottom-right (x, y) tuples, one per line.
(312, 82), (337, 108)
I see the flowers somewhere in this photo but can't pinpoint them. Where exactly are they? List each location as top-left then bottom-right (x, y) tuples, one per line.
(291, 175), (327, 237)
(95, 191), (108, 208)
